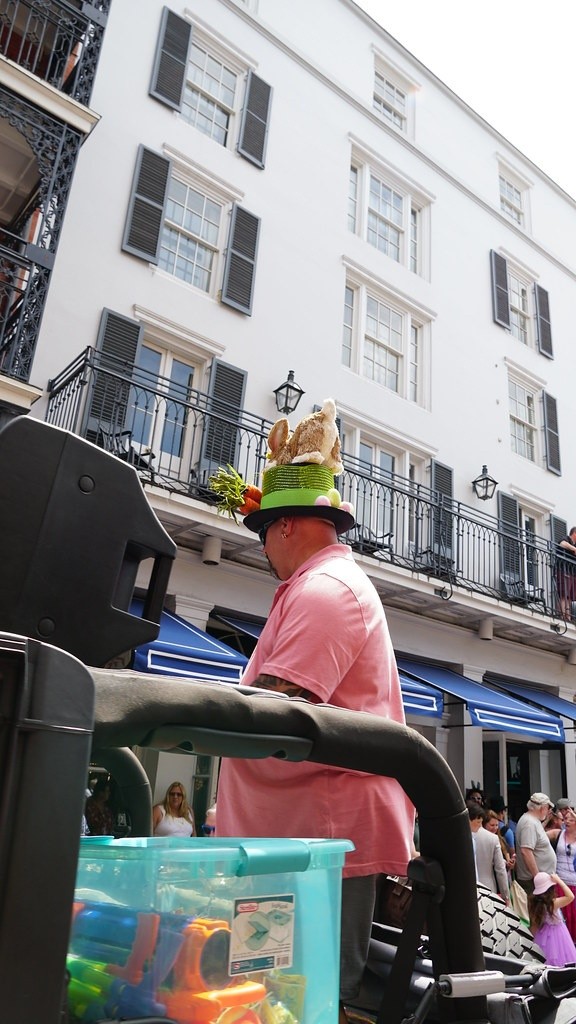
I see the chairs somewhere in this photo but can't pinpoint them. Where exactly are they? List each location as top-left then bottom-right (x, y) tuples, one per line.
(500, 570), (548, 613)
(409, 542), (464, 584)
(190, 455), (233, 507)
(347, 523), (396, 561)
(97, 421), (157, 481)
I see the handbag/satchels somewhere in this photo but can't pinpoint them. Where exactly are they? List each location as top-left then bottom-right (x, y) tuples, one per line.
(510, 881), (530, 921)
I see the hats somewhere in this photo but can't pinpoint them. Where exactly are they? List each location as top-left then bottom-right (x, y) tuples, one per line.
(557, 798), (574, 810)
(489, 796), (508, 813)
(533, 872), (556, 894)
(530, 793), (555, 808)
(242, 462), (354, 533)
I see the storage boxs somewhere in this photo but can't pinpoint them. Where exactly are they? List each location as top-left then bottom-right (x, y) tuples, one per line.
(61, 831), (357, 1023)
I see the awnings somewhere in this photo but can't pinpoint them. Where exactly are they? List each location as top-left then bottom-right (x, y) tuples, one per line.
(128, 599), (250, 684)
(484, 676), (576, 729)
(211, 613), (443, 718)
(397, 655), (565, 741)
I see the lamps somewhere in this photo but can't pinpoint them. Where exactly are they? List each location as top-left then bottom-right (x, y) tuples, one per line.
(568, 648), (576, 665)
(201, 535), (220, 565)
(272, 370), (305, 416)
(479, 619), (494, 639)
(472, 464), (499, 500)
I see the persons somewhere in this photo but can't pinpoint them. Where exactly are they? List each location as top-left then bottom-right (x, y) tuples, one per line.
(555, 526), (576, 621)
(153, 781), (196, 837)
(466, 789), (575, 967)
(86, 779), (115, 834)
(216, 462), (421, 1007)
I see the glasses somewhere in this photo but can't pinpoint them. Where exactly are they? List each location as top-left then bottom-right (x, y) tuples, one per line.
(259, 518), (278, 544)
(566, 844), (571, 855)
(169, 792), (183, 796)
(471, 796), (482, 800)
